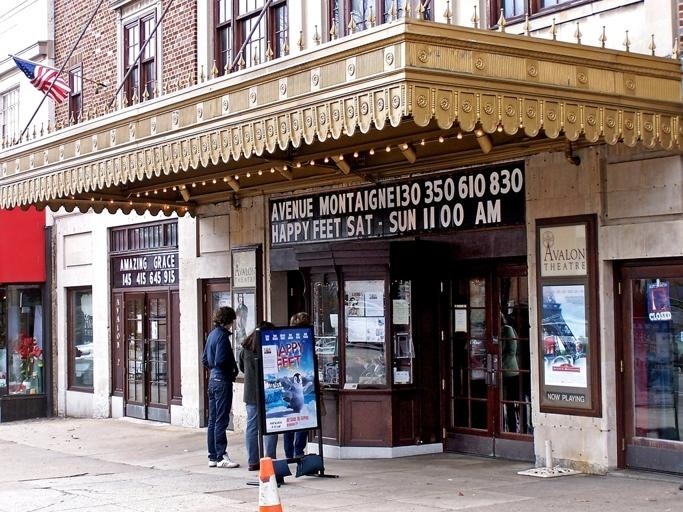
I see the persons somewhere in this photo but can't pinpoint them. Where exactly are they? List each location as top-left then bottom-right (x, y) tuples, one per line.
(236, 293), (248, 350)
(201, 306), (241, 469)
(283, 311), (327, 459)
(462, 322), (487, 429)
(239, 321), (280, 472)
(501, 311), (521, 433)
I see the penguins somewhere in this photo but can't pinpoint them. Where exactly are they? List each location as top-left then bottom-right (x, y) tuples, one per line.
(282, 372), (313, 410)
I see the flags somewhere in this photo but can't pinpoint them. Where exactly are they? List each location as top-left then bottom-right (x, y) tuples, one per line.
(11, 57), (71, 105)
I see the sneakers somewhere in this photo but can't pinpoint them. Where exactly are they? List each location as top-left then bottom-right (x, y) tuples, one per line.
(209, 452), (261, 470)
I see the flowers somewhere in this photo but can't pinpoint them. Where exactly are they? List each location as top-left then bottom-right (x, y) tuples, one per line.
(15, 335), (44, 379)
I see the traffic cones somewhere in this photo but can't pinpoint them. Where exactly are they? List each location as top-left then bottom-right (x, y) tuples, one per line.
(258, 457), (282, 512)
